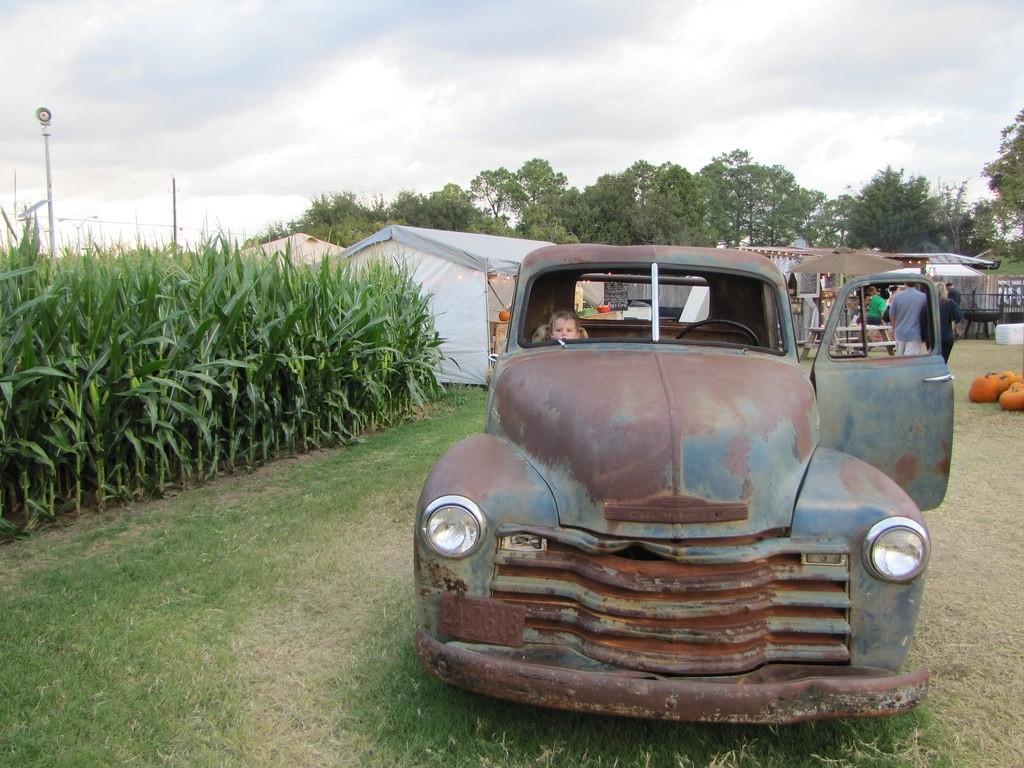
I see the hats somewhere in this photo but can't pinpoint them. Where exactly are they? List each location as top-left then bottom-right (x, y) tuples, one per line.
(946, 283), (953, 287)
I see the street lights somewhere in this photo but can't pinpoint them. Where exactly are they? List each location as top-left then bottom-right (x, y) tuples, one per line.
(58, 215), (99, 255)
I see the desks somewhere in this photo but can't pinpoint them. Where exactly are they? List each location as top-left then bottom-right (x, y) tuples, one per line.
(798, 324), (895, 359)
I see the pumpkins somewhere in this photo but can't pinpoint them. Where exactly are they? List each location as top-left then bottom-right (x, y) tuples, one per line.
(499, 309), (510, 321)
(968, 371), (1024, 411)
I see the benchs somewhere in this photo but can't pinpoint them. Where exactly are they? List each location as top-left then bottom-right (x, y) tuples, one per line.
(778, 336), (861, 343)
(805, 340), (896, 348)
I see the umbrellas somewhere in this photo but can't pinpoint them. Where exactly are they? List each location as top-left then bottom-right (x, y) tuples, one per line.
(784, 247), (905, 354)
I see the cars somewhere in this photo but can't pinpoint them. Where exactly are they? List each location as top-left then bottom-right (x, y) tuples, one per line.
(411, 242), (955, 727)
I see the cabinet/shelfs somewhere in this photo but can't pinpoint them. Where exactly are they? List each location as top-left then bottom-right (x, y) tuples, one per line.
(488, 320), (509, 356)
(582, 310), (624, 320)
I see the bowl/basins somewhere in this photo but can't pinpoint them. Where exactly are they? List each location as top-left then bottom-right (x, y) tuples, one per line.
(597, 306), (610, 312)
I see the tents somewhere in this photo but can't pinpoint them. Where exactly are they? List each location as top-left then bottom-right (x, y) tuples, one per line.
(311, 225), (558, 386)
(240, 232), (347, 271)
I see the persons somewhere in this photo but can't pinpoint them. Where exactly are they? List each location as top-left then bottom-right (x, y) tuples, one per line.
(864, 282), (963, 366)
(532, 310), (590, 342)
